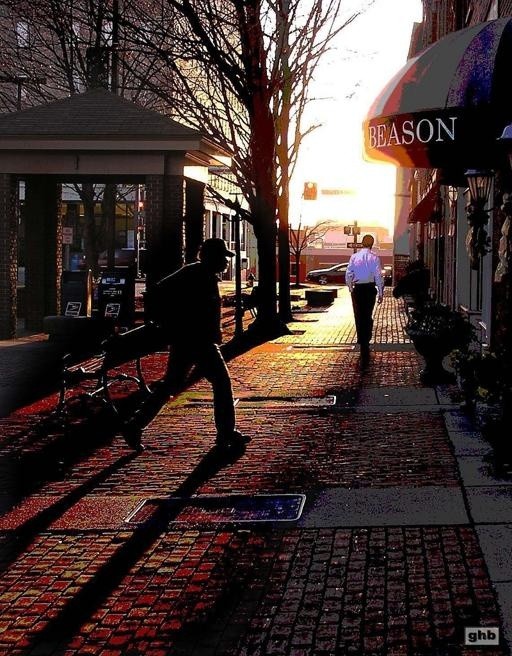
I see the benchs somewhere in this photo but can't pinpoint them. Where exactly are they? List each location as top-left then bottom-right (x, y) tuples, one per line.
(53, 315), (177, 427)
(220, 285), (259, 330)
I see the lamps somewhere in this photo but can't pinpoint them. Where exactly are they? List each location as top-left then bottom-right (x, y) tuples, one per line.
(429, 182), (449, 226)
(457, 159), (501, 255)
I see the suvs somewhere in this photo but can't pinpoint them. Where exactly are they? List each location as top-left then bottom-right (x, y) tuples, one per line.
(94, 247), (146, 271)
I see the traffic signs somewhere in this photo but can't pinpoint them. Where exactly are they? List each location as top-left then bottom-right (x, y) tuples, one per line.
(346, 242), (366, 248)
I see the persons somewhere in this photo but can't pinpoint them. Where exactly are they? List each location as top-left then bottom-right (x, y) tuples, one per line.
(344, 234), (384, 359)
(121, 237), (253, 449)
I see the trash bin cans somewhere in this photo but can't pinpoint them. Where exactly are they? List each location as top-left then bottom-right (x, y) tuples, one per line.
(60, 266), (135, 320)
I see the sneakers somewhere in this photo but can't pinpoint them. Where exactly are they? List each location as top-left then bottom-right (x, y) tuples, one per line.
(121, 423), (145, 451)
(214, 428), (252, 445)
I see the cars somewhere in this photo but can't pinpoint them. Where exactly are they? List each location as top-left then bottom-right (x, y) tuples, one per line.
(305, 260), (393, 285)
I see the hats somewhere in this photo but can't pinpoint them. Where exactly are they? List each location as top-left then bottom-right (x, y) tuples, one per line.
(202, 239), (235, 257)
